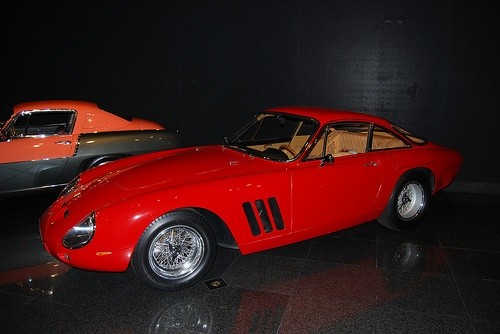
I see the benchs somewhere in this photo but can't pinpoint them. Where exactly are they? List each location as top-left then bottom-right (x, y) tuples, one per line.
(332, 131), (392, 156)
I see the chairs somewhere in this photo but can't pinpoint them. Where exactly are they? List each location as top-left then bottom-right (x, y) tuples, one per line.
(283, 135), (310, 159)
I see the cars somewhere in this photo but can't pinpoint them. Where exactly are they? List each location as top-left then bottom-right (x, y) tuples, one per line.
(0, 102), (182, 209)
(37, 106), (468, 292)
